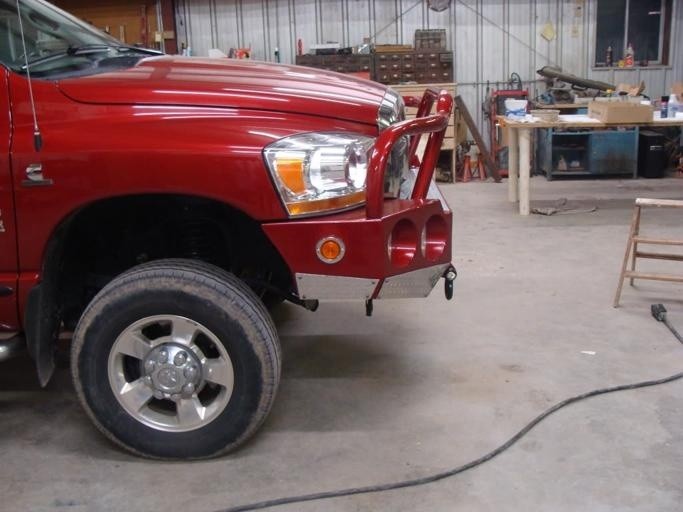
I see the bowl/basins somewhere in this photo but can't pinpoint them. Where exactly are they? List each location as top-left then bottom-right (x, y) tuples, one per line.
(529, 109), (560, 123)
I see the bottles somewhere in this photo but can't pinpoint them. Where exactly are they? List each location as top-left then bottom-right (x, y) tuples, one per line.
(667, 94), (678, 119)
(618, 58), (624, 69)
(181, 46), (191, 56)
(274, 48), (280, 64)
(605, 46), (612, 67)
(660, 95), (669, 118)
(624, 42), (634, 68)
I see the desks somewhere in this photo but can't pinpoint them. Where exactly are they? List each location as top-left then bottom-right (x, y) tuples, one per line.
(494, 105), (683, 218)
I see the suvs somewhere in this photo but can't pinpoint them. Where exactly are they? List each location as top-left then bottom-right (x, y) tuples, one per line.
(0, 1), (456, 461)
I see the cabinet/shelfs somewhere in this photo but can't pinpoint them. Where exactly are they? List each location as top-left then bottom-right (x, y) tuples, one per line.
(536, 104), (641, 181)
(390, 82), (468, 184)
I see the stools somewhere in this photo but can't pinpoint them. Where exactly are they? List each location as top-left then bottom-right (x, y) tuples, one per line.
(611, 196), (683, 310)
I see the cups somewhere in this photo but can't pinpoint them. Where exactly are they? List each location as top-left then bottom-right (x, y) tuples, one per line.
(363, 38), (369, 44)
(642, 58), (648, 67)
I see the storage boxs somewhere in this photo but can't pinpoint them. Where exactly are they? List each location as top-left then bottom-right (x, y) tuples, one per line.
(295, 28), (455, 84)
(587, 101), (654, 127)
